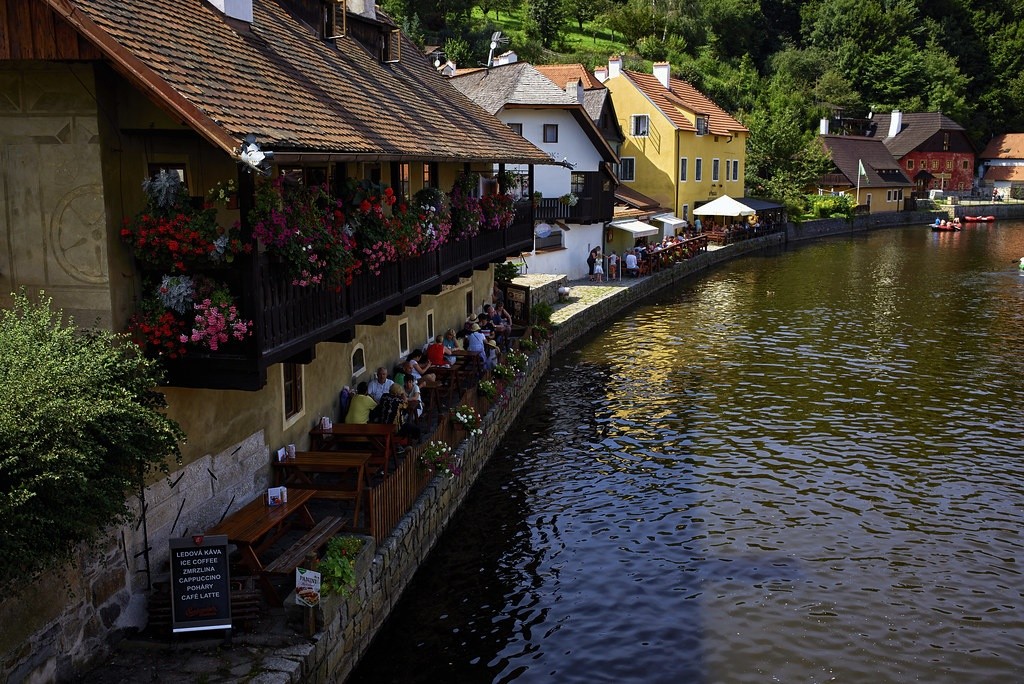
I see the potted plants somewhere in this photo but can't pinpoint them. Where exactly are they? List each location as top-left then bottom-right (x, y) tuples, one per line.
(531, 325), (549, 344)
(533, 299), (555, 327)
(283, 533), (377, 630)
(528, 191), (542, 208)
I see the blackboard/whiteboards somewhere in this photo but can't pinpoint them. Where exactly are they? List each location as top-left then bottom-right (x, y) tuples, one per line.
(168, 535), (232, 629)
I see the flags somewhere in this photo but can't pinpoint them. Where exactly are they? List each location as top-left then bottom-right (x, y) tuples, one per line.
(860, 160), (870, 184)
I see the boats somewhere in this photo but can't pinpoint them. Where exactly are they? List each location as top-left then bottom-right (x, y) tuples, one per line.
(965, 215), (994, 222)
(1018, 263), (1024, 270)
(929, 223), (962, 231)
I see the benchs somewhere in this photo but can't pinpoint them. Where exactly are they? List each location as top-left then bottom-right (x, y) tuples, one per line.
(312, 491), (363, 530)
(325, 435), (404, 468)
(617, 267), (646, 279)
(259, 515), (353, 598)
(371, 456), (389, 483)
(458, 371), (475, 388)
(297, 465), (380, 489)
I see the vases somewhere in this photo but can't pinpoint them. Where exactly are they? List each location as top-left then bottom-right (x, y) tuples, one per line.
(454, 421), (464, 431)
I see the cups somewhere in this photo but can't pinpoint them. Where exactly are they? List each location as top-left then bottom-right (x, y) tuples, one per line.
(288, 444), (295, 459)
(322, 416), (330, 430)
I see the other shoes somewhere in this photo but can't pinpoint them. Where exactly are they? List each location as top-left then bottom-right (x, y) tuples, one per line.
(397, 445), (404, 453)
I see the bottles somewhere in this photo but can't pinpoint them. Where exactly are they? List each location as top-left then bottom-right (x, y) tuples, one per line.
(330, 421), (332, 428)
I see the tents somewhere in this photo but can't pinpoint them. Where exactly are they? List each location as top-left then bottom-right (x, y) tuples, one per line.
(690, 194), (756, 228)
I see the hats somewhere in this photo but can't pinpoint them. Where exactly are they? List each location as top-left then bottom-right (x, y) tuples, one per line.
(470, 323), (481, 331)
(468, 314), (479, 323)
(488, 340), (496, 348)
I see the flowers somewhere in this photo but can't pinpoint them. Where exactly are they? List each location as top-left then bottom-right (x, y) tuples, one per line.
(453, 172), (479, 197)
(416, 440), (461, 480)
(558, 192), (579, 207)
(519, 335), (540, 353)
(477, 379), (504, 403)
(496, 170), (520, 192)
(444, 185), (486, 242)
(449, 404), (484, 436)
(506, 348), (529, 369)
(481, 177), (497, 184)
(493, 363), (526, 384)
(481, 191), (527, 232)
(117, 133), (453, 359)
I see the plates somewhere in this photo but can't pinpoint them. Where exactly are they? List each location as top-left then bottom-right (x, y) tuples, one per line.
(297, 590), (320, 607)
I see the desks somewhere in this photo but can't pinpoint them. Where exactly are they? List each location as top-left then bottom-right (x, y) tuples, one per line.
(452, 351), (483, 388)
(430, 368), (462, 409)
(271, 450), (373, 530)
(638, 261), (647, 277)
(207, 487), (319, 608)
(406, 400), (420, 442)
(309, 423), (400, 482)
(427, 384), (443, 419)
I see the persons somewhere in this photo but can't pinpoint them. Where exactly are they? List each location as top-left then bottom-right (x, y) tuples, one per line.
(377, 383), (421, 454)
(493, 301), (512, 337)
(403, 349), (447, 409)
(490, 280), (504, 309)
(464, 313), (479, 334)
(934, 215), (961, 230)
(465, 323), (489, 364)
(442, 328), (460, 365)
(369, 367), (395, 404)
(426, 335), (453, 365)
(477, 313), (496, 358)
(400, 373), (423, 423)
(587, 217), (744, 283)
(485, 339), (501, 368)
(483, 304), (504, 346)
(393, 361), (426, 396)
(345, 381), (377, 425)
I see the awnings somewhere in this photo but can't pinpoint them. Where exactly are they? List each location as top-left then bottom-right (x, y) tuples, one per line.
(608, 219), (659, 238)
(732, 197), (780, 211)
(654, 215), (687, 229)
